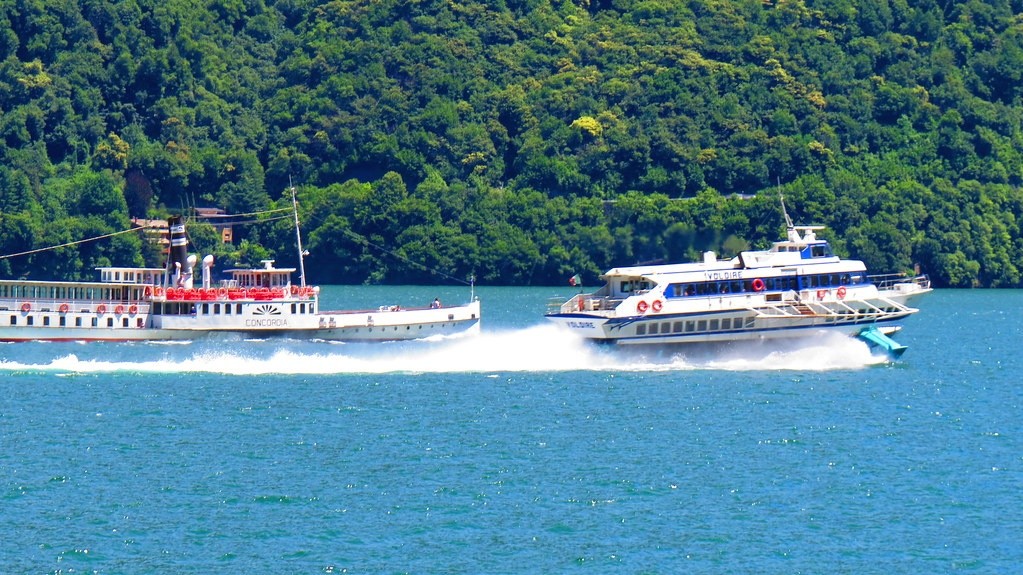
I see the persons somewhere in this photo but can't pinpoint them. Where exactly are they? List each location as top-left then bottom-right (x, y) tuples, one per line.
(396, 306), (400, 311)
(698, 284), (706, 295)
(712, 283), (739, 293)
(241, 284), (245, 288)
(432, 298), (439, 309)
(684, 285), (694, 296)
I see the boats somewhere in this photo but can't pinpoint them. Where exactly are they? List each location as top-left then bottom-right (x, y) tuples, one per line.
(543, 175), (933, 365)
(0, 176), (479, 346)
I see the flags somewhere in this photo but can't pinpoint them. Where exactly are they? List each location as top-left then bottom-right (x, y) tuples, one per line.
(569, 273), (581, 286)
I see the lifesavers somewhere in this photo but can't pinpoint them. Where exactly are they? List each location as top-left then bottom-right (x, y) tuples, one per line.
(218, 287), (226, 297)
(652, 300), (663, 311)
(22, 303), (30, 312)
(838, 286), (847, 298)
(115, 305), (123, 314)
(637, 300), (648, 312)
(145, 286), (151, 296)
(177, 287), (184, 294)
(208, 288), (216, 294)
(752, 279), (764, 292)
(129, 305), (137, 314)
(230, 287), (279, 293)
(188, 288), (195, 293)
(60, 304), (69, 313)
(198, 287), (205, 293)
(98, 305), (106, 314)
(167, 287), (175, 295)
(292, 285), (313, 296)
(155, 287), (163, 296)
